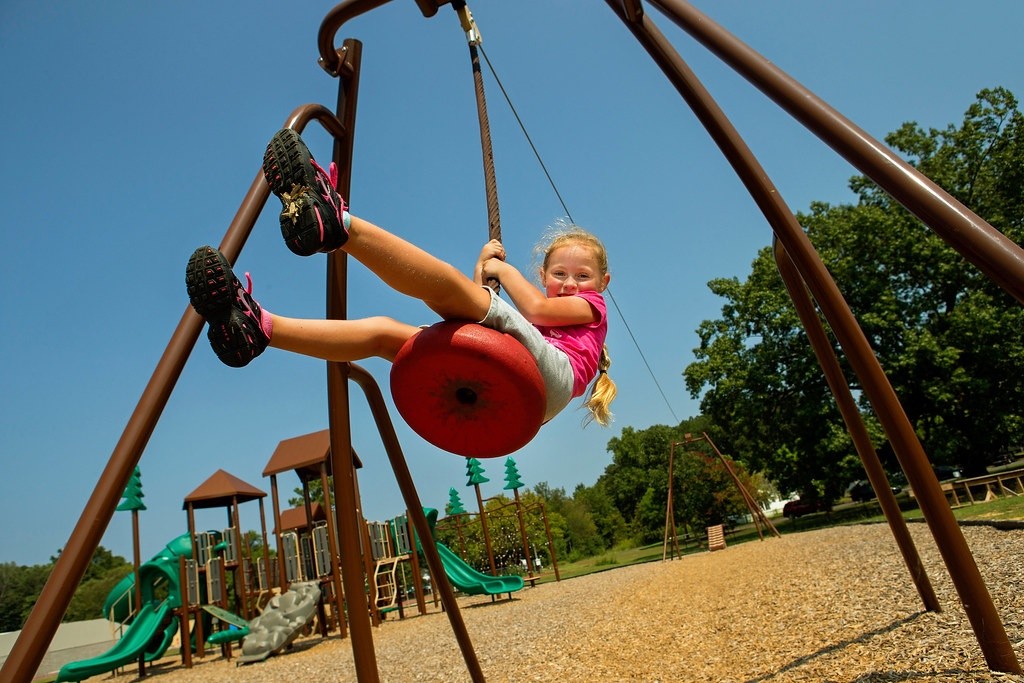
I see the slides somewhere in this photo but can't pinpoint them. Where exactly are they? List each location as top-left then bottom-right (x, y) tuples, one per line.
(55, 604), (170, 683)
(103, 532), (193, 662)
(436, 542), (524, 595)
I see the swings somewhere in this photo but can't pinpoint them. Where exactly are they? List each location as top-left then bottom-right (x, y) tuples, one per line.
(390, 30), (547, 458)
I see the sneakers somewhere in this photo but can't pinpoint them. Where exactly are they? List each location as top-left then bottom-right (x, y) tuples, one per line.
(263, 127), (350, 256)
(186, 245), (271, 367)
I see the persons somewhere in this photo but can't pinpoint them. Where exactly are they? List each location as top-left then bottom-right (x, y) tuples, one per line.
(187, 129), (619, 431)
